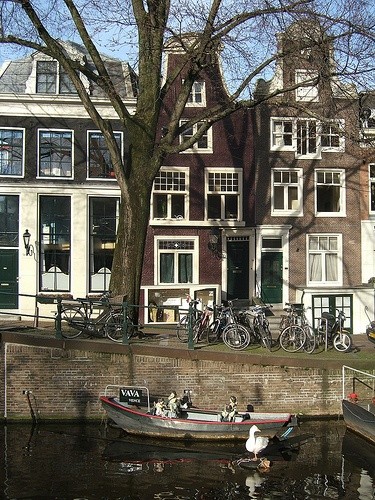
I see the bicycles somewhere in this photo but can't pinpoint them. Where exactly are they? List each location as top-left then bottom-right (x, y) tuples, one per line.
(176, 293), (317, 354)
(54, 290), (134, 343)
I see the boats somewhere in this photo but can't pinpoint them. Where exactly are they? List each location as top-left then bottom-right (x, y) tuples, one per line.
(102, 432), (301, 465)
(99, 393), (292, 439)
(340, 430), (375, 476)
(342, 398), (375, 442)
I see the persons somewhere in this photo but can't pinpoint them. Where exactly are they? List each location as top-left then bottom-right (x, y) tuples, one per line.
(155, 398), (167, 418)
(221, 395), (238, 422)
(168, 390), (181, 414)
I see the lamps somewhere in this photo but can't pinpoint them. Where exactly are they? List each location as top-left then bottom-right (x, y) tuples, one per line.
(23, 230), (33, 256)
(209, 230), (218, 252)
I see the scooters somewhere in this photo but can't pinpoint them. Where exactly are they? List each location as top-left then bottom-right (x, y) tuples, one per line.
(320, 306), (353, 353)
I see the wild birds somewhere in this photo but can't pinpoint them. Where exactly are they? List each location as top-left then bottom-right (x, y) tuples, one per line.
(244, 424), (271, 463)
